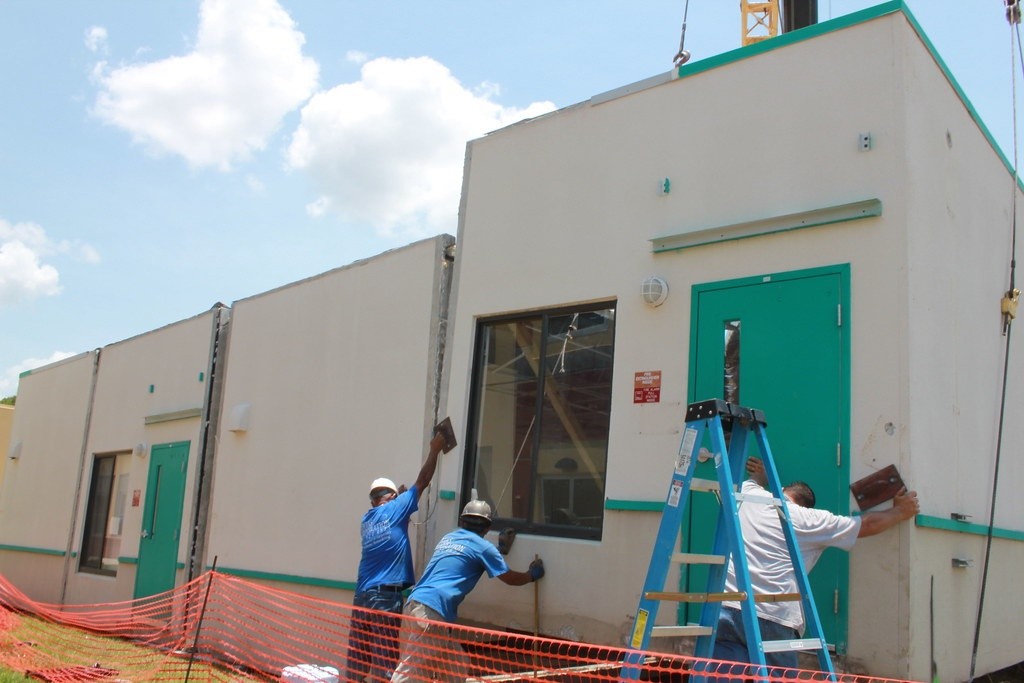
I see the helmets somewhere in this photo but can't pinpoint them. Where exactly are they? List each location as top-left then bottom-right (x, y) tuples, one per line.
(368, 477), (399, 497)
(460, 499), (494, 523)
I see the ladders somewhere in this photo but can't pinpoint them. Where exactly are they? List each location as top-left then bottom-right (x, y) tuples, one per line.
(617, 398), (837, 683)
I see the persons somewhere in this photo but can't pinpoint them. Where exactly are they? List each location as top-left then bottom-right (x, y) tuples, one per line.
(344, 431), (446, 683)
(390, 500), (545, 683)
(708, 456), (920, 683)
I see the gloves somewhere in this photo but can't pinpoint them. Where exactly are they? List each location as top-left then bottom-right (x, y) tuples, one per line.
(497, 528), (517, 555)
(528, 559), (546, 582)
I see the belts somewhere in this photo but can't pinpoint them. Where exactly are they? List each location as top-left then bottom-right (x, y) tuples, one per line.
(370, 585), (403, 590)
